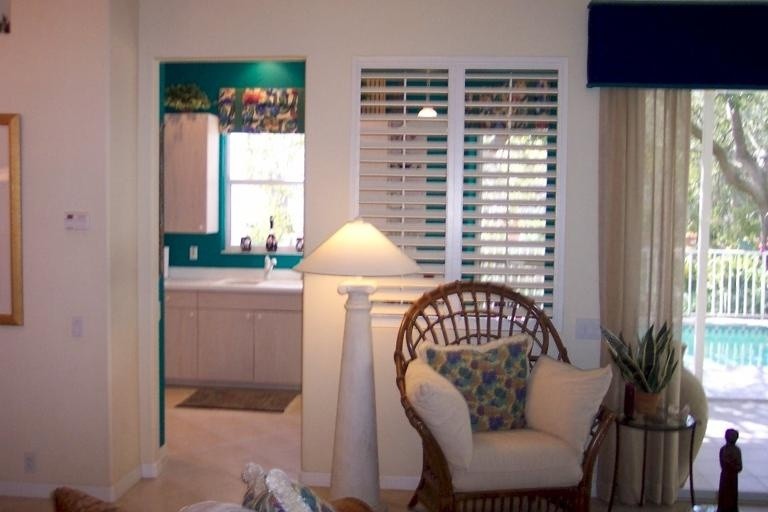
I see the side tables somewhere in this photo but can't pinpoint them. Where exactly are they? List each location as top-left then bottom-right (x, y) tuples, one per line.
(607, 407), (702, 512)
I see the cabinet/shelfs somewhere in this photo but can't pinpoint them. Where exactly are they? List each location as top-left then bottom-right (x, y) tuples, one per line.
(162, 113), (221, 235)
(163, 290), (303, 394)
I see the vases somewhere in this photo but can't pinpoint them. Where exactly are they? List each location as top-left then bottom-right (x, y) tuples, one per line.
(674, 338), (709, 491)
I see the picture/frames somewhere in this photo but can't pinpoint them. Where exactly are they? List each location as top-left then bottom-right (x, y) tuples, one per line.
(0, 111), (25, 327)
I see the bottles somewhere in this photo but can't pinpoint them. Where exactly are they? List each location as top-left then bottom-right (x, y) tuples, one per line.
(295, 238), (304, 252)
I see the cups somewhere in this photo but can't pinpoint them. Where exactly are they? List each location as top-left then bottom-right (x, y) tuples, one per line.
(666, 401), (690, 427)
(240, 237), (251, 252)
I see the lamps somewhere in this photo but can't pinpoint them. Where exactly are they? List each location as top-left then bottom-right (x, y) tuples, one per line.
(290, 215), (423, 512)
(416, 69), (437, 118)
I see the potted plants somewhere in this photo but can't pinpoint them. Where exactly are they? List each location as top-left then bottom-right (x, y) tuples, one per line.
(164, 80), (211, 114)
(599, 320), (679, 414)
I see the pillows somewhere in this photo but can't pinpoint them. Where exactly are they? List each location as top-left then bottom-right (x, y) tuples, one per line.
(405, 330), (613, 481)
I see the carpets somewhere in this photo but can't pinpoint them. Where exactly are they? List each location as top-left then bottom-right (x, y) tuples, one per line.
(175, 386), (300, 413)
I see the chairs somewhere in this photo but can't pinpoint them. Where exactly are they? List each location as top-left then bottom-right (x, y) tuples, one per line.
(393, 281), (618, 512)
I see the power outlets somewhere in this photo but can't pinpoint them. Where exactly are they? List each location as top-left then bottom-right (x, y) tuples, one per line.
(189, 245), (198, 261)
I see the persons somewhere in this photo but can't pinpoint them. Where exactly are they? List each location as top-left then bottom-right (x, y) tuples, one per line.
(716, 427), (742, 512)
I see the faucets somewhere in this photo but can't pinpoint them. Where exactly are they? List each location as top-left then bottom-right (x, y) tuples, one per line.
(263, 256), (277, 278)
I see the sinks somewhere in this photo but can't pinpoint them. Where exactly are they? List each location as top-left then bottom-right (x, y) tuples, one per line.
(218, 279), (262, 286)
